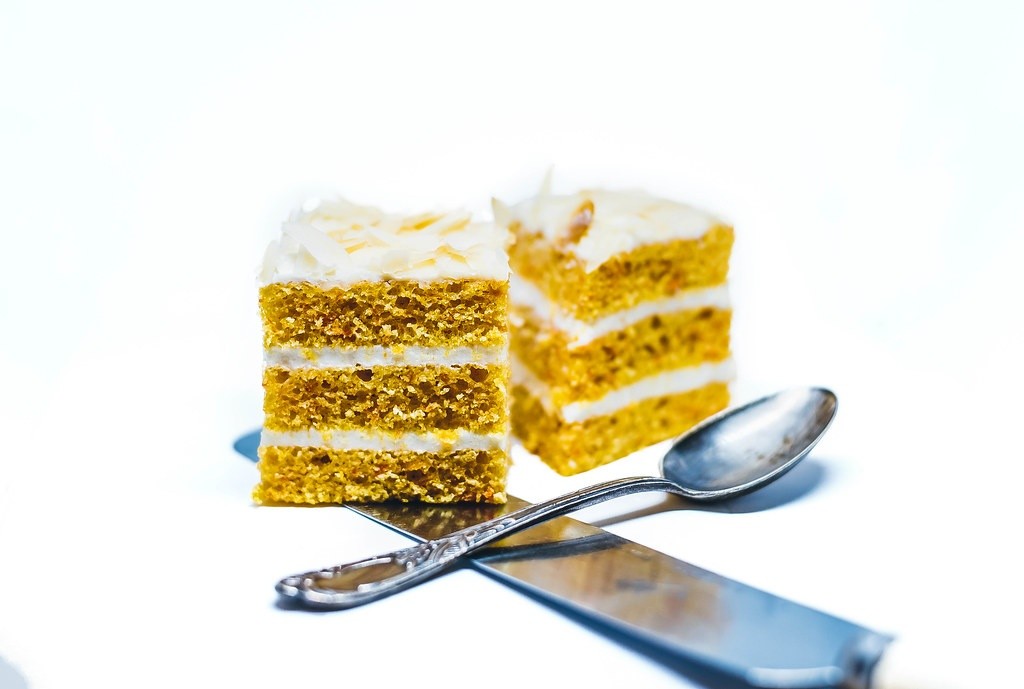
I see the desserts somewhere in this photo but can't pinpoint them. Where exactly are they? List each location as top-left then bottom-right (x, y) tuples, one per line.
(252, 203), (513, 505)
(488, 184), (737, 473)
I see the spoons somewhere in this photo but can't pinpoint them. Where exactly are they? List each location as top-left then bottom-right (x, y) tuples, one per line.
(275, 384), (839, 610)
